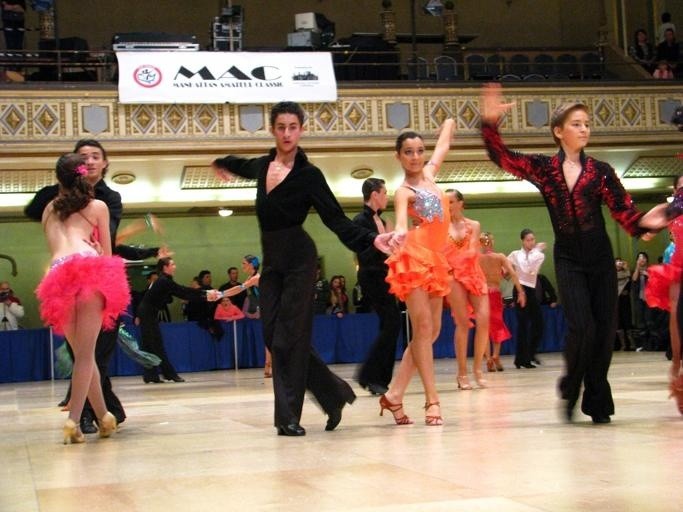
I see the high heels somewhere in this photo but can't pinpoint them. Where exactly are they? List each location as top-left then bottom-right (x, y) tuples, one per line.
(457, 356), (535, 390)
(99, 412), (116, 437)
(64, 419), (85, 444)
(425, 402), (444, 425)
(380, 394), (415, 424)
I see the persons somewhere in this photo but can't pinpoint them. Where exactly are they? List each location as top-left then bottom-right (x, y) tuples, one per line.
(656, 12), (677, 45)
(207, 95), (407, 437)
(310, 178), (403, 396)
(377, 118), (460, 427)
(35, 151), (132, 448)
(0, 1), (26, 72)
(0, 282), (24, 331)
(59, 244), (273, 411)
(475, 80), (675, 427)
(651, 56), (674, 80)
(443, 189), (556, 391)
(614, 231), (683, 361)
(656, 29), (682, 81)
(21, 139), (127, 437)
(628, 28), (658, 76)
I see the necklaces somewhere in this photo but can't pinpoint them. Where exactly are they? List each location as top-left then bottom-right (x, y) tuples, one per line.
(273, 155), (290, 171)
(564, 159), (580, 170)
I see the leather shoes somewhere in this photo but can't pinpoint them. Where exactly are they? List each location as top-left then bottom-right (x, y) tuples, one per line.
(80, 406), (95, 433)
(144, 375), (184, 383)
(278, 424), (305, 435)
(326, 382), (357, 430)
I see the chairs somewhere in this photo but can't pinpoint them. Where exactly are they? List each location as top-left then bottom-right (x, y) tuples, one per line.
(411, 54), (606, 83)
